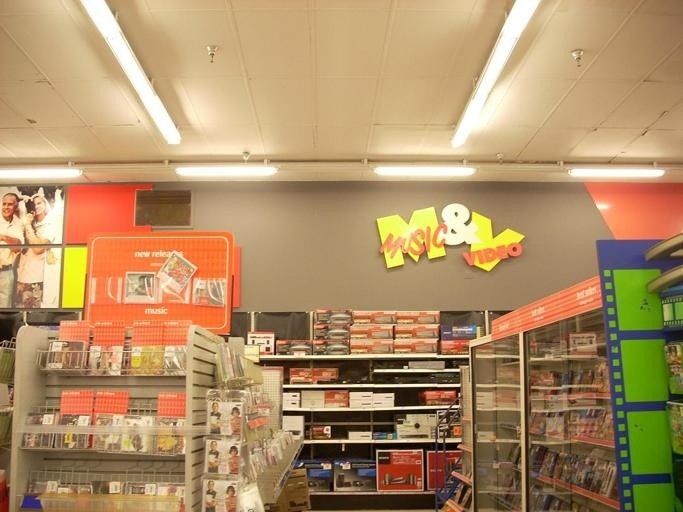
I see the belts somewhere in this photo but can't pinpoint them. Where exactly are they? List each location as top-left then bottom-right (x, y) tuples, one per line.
(0, 267), (12, 271)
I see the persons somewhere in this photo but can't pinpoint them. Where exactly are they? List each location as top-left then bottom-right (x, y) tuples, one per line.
(666, 346), (682, 366)
(134, 276), (146, 295)
(0, 193), (24, 308)
(11, 193), (55, 308)
(204, 402), (242, 511)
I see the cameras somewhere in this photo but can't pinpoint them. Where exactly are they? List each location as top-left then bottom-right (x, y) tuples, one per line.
(25, 199), (35, 214)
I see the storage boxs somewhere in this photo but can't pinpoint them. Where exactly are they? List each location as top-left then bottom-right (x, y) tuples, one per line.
(247, 310), (465, 492)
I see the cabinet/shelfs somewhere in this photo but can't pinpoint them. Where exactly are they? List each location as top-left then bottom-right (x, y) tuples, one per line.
(466, 302), (621, 510)
